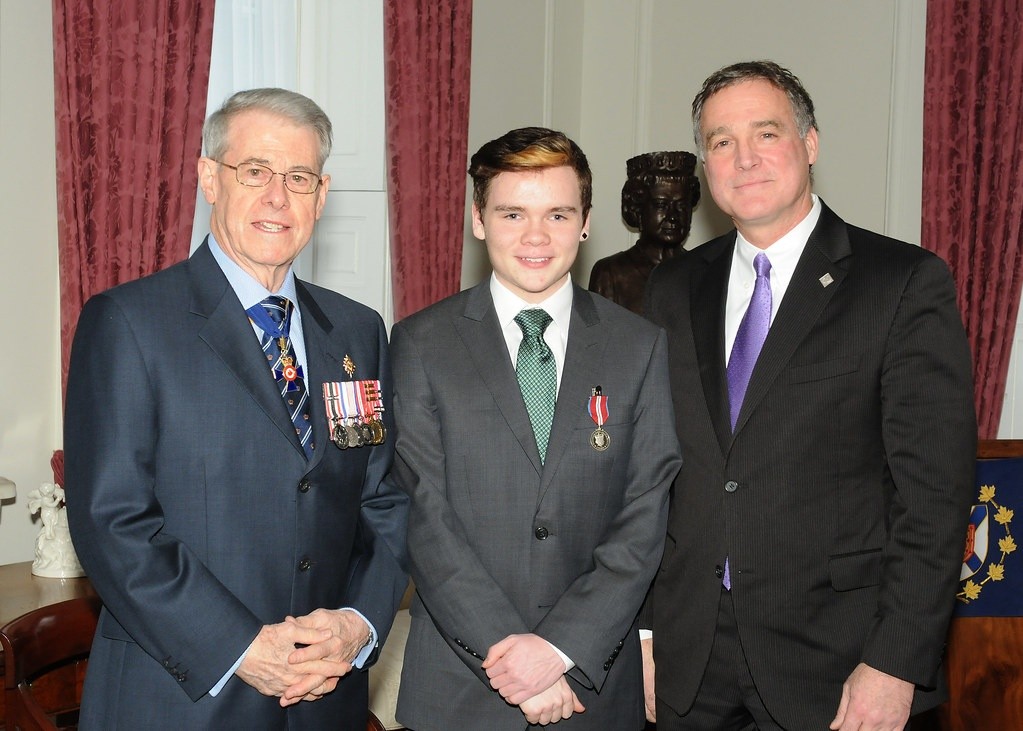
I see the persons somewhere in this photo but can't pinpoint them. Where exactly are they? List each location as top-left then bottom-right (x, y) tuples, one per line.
(393, 126), (686, 731)
(588, 149), (701, 311)
(62, 86), (411, 731)
(634, 59), (980, 730)
(40, 482), (63, 539)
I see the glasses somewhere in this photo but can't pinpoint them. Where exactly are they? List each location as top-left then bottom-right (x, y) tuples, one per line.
(211, 157), (323, 194)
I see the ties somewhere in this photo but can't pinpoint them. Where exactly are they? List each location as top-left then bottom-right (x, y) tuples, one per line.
(722, 252), (771, 591)
(514, 308), (557, 467)
(256, 295), (316, 462)
